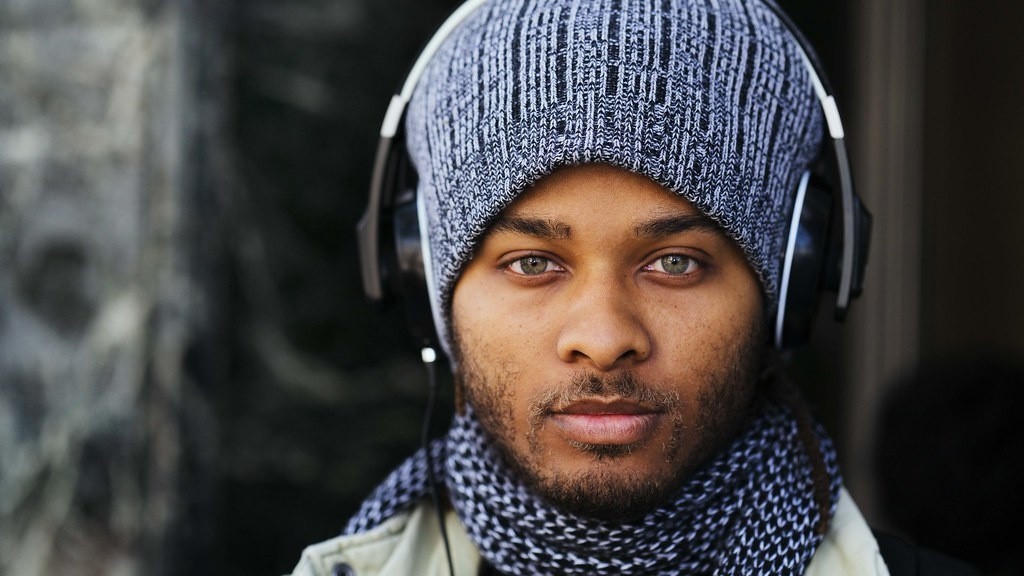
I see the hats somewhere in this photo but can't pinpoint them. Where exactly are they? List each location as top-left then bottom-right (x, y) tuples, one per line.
(405, 0), (824, 363)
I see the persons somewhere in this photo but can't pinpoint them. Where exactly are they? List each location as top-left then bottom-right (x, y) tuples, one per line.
(290, 0), (890, 576)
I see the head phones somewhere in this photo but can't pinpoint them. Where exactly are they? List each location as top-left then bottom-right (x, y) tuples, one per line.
(358, 0), (873, 365)
